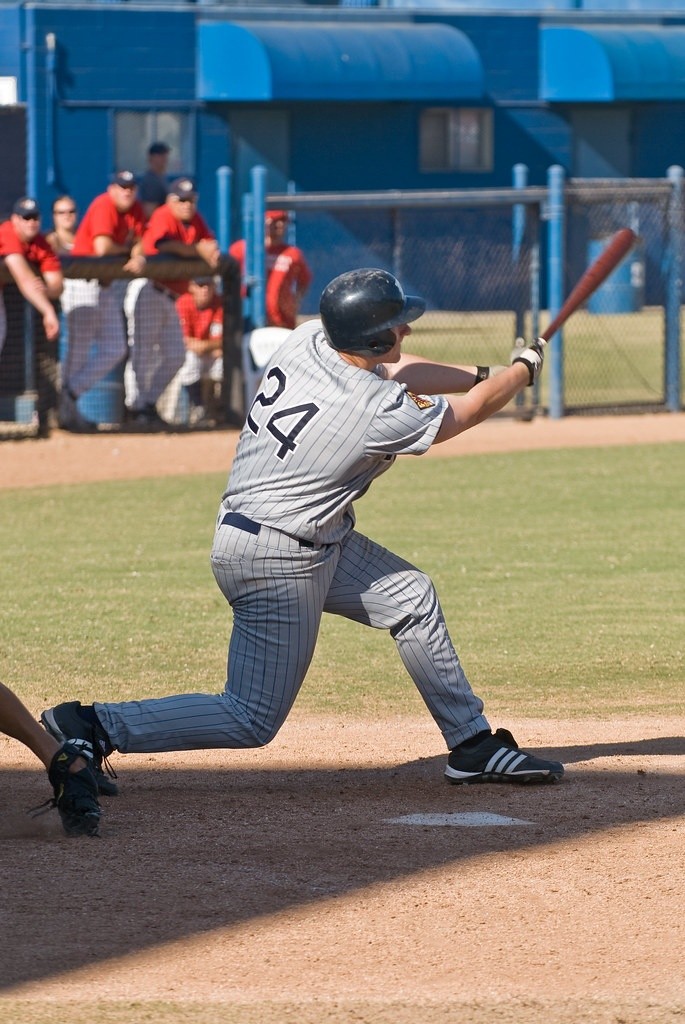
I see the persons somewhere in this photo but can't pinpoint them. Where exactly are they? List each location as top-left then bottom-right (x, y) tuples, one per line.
(41, 269), (565, 795)
(133, 141), (174, 216)
(1, 682), (117, 840)
(0, 168), (314, 431)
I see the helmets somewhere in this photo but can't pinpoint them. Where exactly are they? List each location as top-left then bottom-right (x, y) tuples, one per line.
(320, 269), (425, 358)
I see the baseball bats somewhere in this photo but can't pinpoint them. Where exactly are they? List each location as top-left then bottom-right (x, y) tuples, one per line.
(536, 227), (638, 346)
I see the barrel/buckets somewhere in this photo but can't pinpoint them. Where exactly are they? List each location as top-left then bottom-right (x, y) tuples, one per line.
(585, 238), (647, 313)
(60, 312), (126, 425)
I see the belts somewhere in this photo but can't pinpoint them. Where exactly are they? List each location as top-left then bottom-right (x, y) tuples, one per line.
(153, 285), (176, 301)
(220, 512), (328, 548)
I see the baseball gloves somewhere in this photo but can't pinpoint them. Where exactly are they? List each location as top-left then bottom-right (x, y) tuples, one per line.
(49, 740), (118, 836)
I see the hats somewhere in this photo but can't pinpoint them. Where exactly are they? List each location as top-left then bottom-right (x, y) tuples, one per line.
(167, 177), (198, 199)
(116, 170), (137, 186)
(150, 143), (169, 155)
(266, 209), (287, 220)
(13, 197), (39, 217)
(194, 277), (215, 286)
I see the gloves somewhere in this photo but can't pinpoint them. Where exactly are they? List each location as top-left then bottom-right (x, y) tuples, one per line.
(511, 338), (544, 387)
(475, 365), (508, 387)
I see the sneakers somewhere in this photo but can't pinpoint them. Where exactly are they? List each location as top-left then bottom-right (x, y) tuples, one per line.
(444, 728), (564, 786)
(43, 700), (119, 797)
